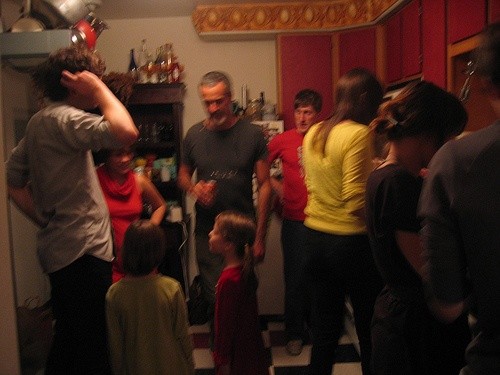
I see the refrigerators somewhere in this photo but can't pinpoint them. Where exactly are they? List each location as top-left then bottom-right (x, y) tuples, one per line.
(250, 120), (285, 316)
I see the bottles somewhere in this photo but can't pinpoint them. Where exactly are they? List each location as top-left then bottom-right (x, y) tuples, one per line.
(131, 122), (177, 202)
(128, 39), (181, 84)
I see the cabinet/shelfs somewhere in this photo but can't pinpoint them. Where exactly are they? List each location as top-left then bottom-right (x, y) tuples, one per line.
(276, 0), (500, 136)
(91, 82), (185, 279)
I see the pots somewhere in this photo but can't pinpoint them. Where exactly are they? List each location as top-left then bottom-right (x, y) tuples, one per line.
(12, 0), (101, 34)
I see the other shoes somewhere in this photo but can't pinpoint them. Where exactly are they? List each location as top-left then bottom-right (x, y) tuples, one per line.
(286, 338), (303, 355)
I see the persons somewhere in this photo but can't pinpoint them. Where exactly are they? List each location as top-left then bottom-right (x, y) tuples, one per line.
(4, 45), (138, 375)
(177, 71), (273, 360)
(207, 212), (271, 375)
(265, 87), (323, 356)
(94, 141), (166, 285)
(104, 221), (195, 375)
(360, 79), (468, 375)
(300, 67), (381, 375)
(417, 21), (500, 375)
